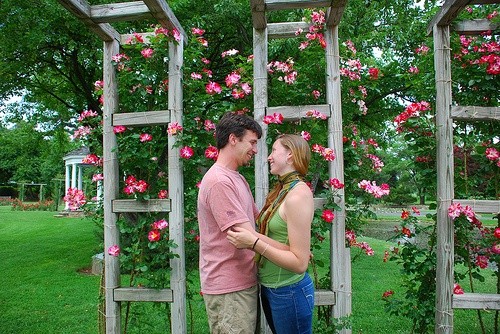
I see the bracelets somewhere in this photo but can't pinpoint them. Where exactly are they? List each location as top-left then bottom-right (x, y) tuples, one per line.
(252, 238), (259, 249)
(261, 245), (270, 255)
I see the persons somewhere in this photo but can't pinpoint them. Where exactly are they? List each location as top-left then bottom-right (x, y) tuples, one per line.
(227, 134), (315, 334)
(198, 113), (314, 334)
